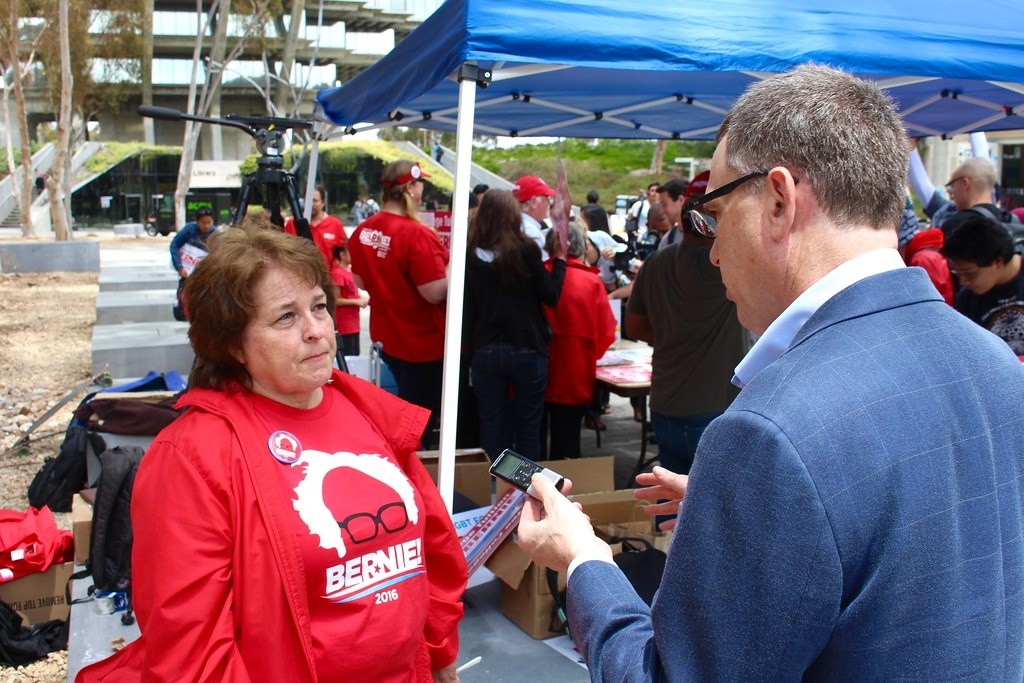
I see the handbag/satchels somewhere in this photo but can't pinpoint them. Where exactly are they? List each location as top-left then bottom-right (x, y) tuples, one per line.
(0, 505), (75, 584)
(546, 537), (669, 643)
(625, 218), (639, 231)
(74, 369), (193, 436)
(0, 599), (70, 668)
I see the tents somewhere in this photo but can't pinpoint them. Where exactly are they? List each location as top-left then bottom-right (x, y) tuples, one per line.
(299, 0), (1024, 527)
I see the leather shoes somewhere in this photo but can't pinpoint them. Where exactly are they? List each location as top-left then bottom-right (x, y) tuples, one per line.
(634, 404), (644, 422)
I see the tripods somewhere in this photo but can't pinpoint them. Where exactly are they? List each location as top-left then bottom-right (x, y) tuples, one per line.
(137, 102), (349, 371)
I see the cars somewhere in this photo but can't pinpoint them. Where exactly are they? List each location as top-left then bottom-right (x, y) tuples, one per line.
(146, 193), (231, 236)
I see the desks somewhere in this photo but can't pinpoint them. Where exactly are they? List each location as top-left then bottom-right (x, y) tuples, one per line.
(68, 265), (592, 683)
(591, 338), (659, 489)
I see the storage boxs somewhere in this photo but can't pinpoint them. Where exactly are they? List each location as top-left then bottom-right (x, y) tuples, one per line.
(71, 493), (95, 566)
(0, 560), (73, 627)
(411, 446), (493, 510)
(485, 455), (673, 641)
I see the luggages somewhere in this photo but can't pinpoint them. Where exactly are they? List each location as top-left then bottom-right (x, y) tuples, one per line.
(367, 340), (404, 397)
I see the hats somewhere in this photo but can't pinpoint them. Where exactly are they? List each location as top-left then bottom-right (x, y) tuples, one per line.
(383, 163), (432, 187)
(511, 173), (556, 202)
(684, 168), (712, 196)
(896, 207), (924, 248)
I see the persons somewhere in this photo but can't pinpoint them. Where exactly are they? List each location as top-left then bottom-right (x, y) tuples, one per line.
(72, 219), (469, 683)
(450, 131), (1024, 533)
(35, 172), (45, 196)
(516, 58), (1024, 683)
(347, 159), (451, 451)
(328, 237), (368, 356)
(169, 206), (222, 324)
(231, 185), (379, 278)
(434, 142), (443, 166)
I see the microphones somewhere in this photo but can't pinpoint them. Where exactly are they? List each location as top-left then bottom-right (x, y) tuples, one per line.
(612, 235), (627, 245)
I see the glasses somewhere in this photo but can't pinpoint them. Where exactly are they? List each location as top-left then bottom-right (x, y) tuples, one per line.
(953, 264), (983, 281)
(195, 209), (213, 219)
(943, 176), (966, 192)
(680, 169), (800, 240)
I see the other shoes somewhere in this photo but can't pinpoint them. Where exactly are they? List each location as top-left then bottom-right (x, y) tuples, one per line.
(585, 416), (607, 430)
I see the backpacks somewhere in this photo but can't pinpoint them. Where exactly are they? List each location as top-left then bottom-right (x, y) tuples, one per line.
(967, 206), (1024, 254)
(85, 444), (145, 604)
(27, 424), (107, 517)
